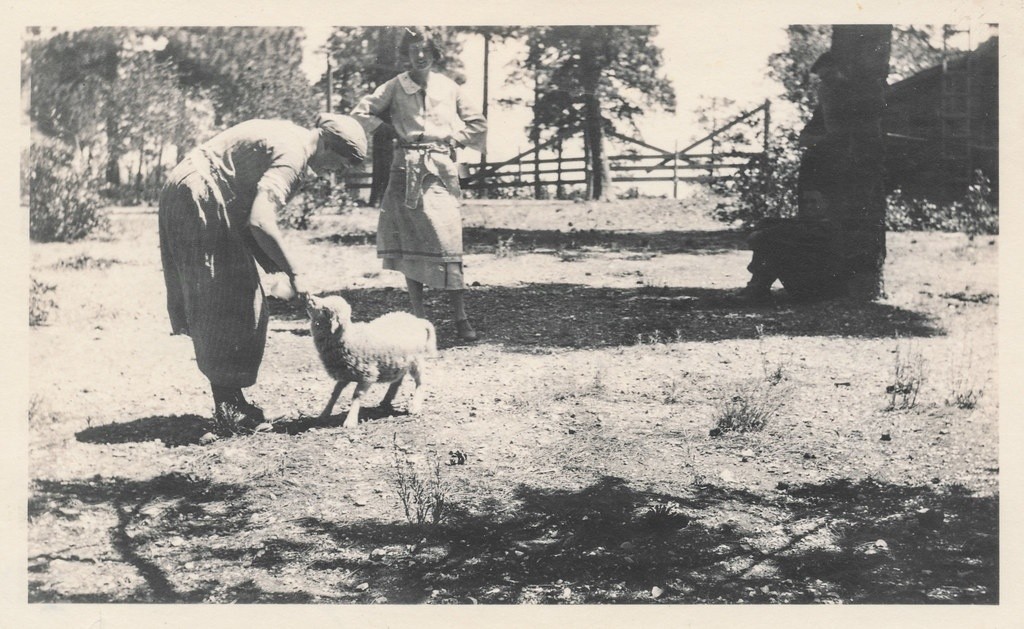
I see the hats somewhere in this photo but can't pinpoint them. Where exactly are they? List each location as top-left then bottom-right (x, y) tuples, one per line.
(315, 112), (367, 168)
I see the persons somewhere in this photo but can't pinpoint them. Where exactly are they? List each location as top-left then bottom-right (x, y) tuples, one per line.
(349, 26), (487, 341)
(727, 179), (851, 306)
(156, 112), (369, 430)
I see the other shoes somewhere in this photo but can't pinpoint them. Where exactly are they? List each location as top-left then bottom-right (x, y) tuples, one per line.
(724, 281), (772, 305)
(457, 317), (477, 342)
(239, 404), (286, 423)
(217, 413), (273, 436)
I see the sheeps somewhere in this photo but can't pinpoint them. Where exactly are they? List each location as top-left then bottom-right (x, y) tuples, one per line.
(307, 294), (437, 427)
